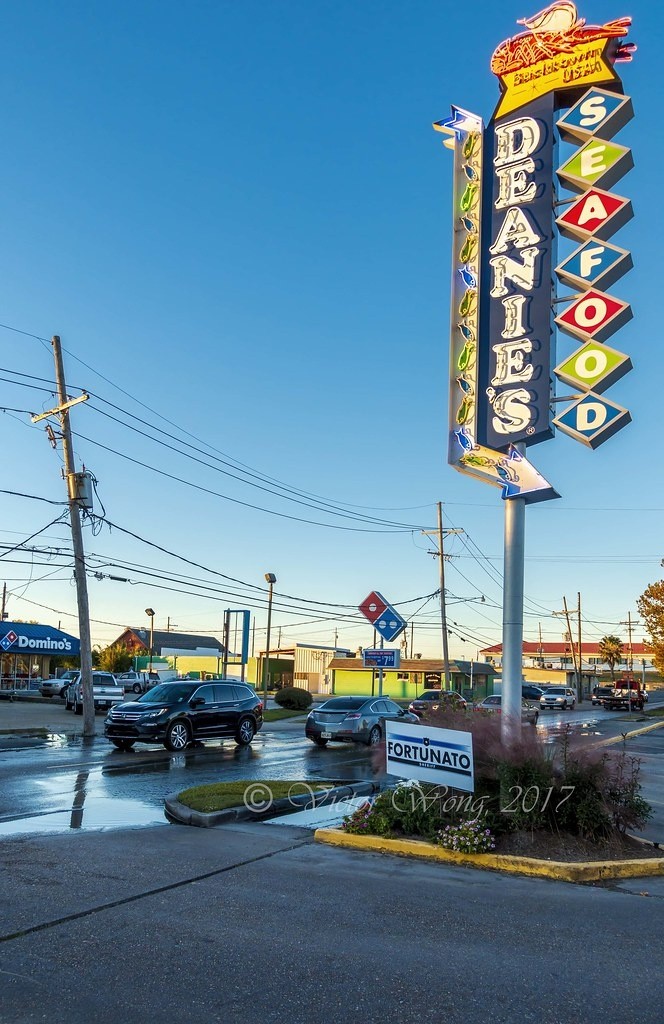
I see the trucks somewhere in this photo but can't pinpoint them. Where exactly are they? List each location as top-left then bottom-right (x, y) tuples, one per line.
(602, 678), (645, 711)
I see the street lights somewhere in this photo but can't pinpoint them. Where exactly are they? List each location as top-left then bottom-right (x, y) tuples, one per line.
(144, 607), (155, 673)
(262, 572), (277, 709)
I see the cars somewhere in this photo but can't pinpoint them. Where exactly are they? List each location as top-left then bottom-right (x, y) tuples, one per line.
(639, 689), (649, 702)
(407, 688), (469, 719)
(537, 686), (578, 711)
(590, 684), (614, 707)
(38, 671), (82, 697)
(473, 694), (540, 728)
(305, 692), (423, 748)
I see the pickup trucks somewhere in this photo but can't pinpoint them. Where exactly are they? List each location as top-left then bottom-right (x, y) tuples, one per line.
(65, 672), (125, 713)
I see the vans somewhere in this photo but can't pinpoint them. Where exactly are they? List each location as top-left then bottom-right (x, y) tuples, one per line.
(116, 671), (161, 692)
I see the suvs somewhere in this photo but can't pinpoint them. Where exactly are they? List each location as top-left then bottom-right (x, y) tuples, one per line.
(103, 679), (264, 752)
